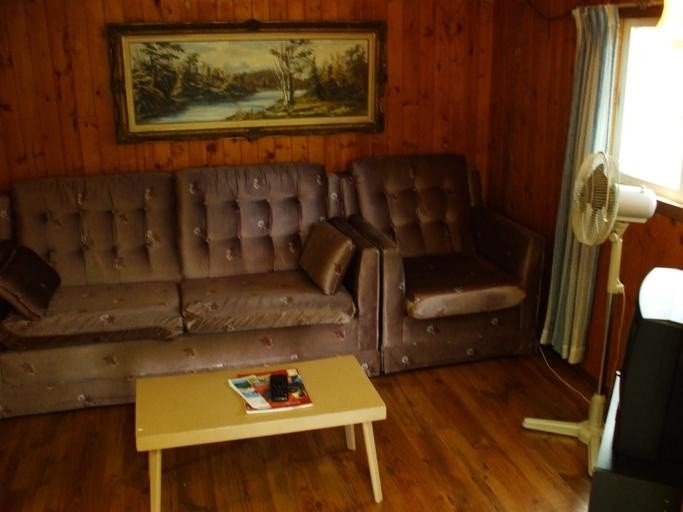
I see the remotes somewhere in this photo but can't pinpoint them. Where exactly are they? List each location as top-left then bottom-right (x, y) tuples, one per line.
(271, 376), (289, 401)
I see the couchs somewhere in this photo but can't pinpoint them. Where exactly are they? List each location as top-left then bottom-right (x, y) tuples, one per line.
(0, 160), (381, 420)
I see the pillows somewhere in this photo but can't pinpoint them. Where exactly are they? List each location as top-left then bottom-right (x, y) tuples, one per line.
(298, 220), (355, 297)
(0, 245), (60, 322)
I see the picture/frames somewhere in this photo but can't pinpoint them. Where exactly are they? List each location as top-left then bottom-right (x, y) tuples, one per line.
(100, 19), (387, 145)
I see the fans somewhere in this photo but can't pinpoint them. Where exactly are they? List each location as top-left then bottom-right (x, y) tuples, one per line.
(520, 146), (657, 481)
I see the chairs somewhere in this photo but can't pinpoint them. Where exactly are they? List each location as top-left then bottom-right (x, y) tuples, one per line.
(346, 153), (545, 375)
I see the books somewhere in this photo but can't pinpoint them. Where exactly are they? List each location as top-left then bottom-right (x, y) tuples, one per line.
(228, 373), (300, 408)
(235, 366), (314, 413)
